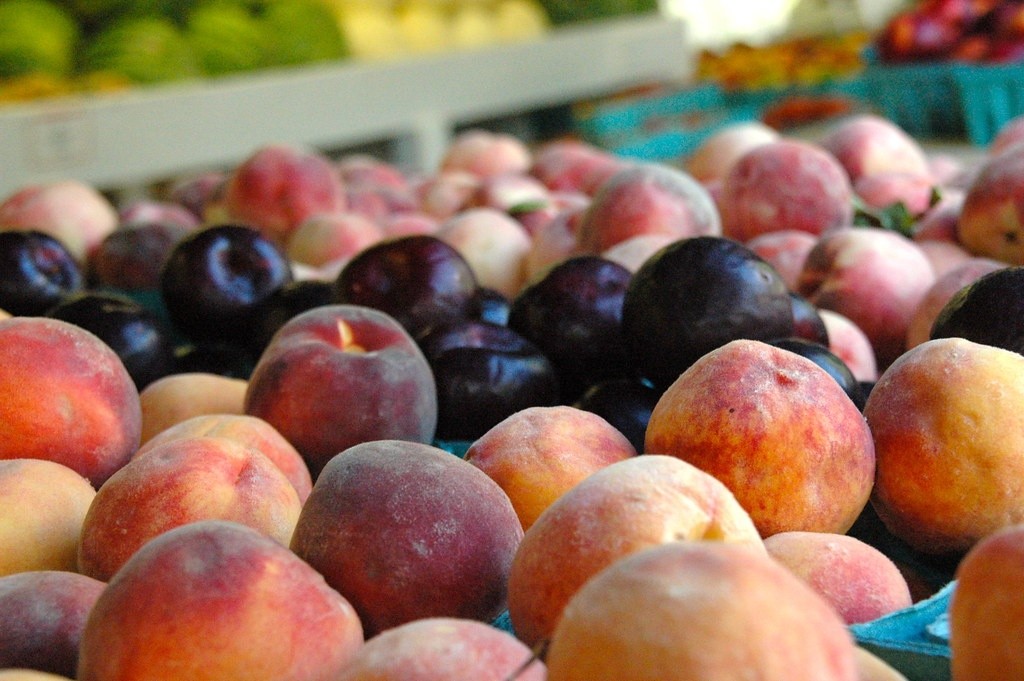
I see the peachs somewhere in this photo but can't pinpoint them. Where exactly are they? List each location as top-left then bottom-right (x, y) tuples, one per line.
(0, 115), (1024, 678)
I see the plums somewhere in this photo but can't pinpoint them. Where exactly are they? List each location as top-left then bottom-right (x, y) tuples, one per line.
(880, 1), (1024, 67)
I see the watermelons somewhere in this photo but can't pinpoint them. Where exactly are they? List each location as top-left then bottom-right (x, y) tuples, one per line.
(0, 0), (361, 98)
